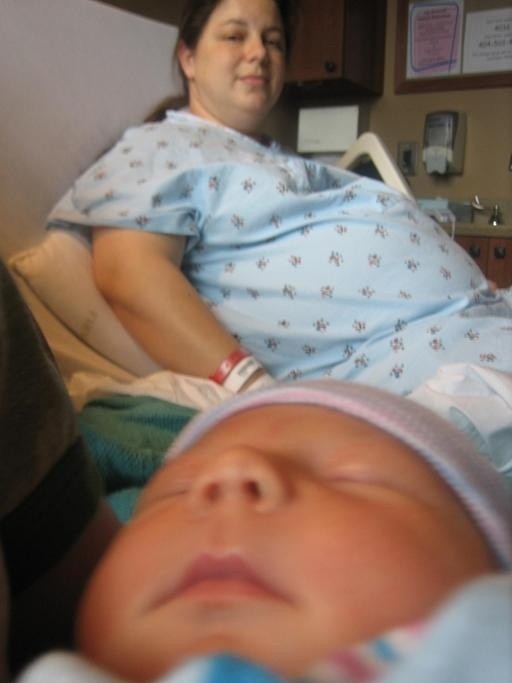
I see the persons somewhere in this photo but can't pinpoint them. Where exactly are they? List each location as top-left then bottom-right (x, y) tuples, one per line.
(1, 258), (122, 683)
(18, 379), (512, 683)
(43, 0), (512, 480)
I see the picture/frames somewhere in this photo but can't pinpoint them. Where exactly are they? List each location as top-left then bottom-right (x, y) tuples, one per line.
(394, 1), (511, 94)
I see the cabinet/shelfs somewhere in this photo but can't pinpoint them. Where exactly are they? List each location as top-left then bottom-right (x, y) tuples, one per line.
(444, 222), (512, 290)
(284, 0), (386, 101)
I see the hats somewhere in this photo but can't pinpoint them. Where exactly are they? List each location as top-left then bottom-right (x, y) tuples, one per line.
(162, 380), (512, 570)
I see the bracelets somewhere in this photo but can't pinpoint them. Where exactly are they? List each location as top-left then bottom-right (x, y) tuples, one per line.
(209, 346), (263, 392)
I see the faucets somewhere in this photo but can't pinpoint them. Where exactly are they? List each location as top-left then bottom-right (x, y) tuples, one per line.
(470, 195), (501, 228)
